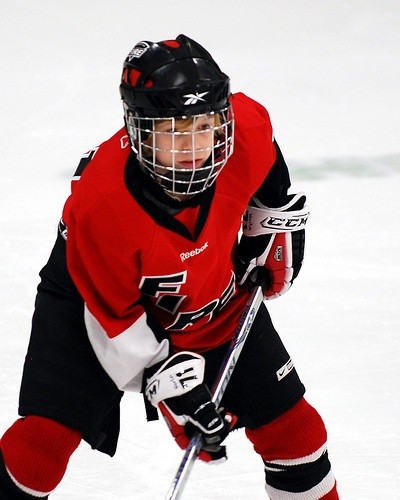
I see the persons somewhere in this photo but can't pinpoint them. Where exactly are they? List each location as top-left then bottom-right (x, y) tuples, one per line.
(0, 33), (347, 500)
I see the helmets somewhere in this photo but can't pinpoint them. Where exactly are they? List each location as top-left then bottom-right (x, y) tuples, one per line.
(118, 34), (231, 143)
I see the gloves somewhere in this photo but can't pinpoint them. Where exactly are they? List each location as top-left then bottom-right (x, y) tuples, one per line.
(235, 193), (311, 301)
(146, 349), (238, 463)
